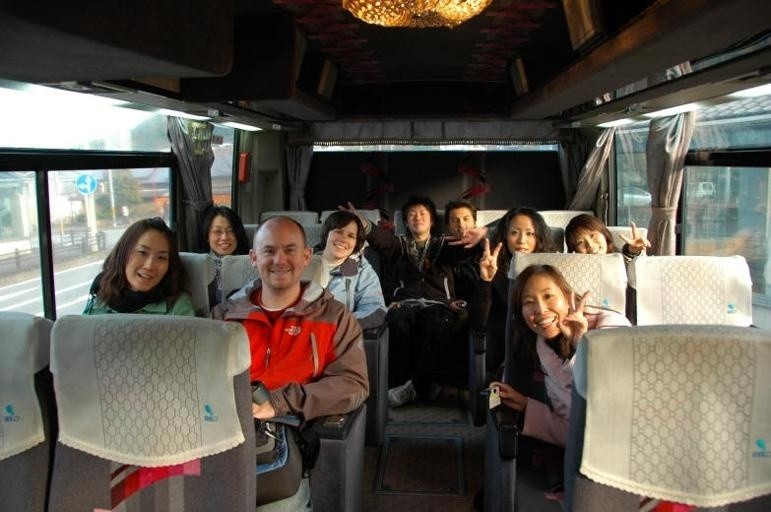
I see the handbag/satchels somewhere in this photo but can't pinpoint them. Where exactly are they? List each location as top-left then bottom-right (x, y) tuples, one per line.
(251, 381), (304, 506)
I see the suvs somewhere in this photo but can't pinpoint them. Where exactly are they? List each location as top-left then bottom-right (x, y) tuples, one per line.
(687, 181), (714, 201)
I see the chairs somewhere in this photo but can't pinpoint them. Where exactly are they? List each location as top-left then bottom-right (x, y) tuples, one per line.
(0, 199), (771, 512)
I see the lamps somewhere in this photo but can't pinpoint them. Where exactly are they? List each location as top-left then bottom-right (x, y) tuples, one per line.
(342, 0), (493, 30)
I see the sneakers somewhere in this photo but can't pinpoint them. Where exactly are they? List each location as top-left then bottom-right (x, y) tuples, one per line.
(388, 380), (416, 407)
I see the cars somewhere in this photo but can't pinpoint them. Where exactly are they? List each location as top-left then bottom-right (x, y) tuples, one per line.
(616, 184), (651, 207)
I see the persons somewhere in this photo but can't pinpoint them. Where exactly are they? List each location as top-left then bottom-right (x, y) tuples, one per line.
(310, 211), (388, 329)
(485, 262), (632, 450)
(337, 194), (468, 409)
(208, 212), (371, 421)
(81, 216), (198, 317)
(564, 212), (652, 264)
(444, 198), (505, 297)
(199, 203), (252, 255)
(469, 204), (562, 296)
